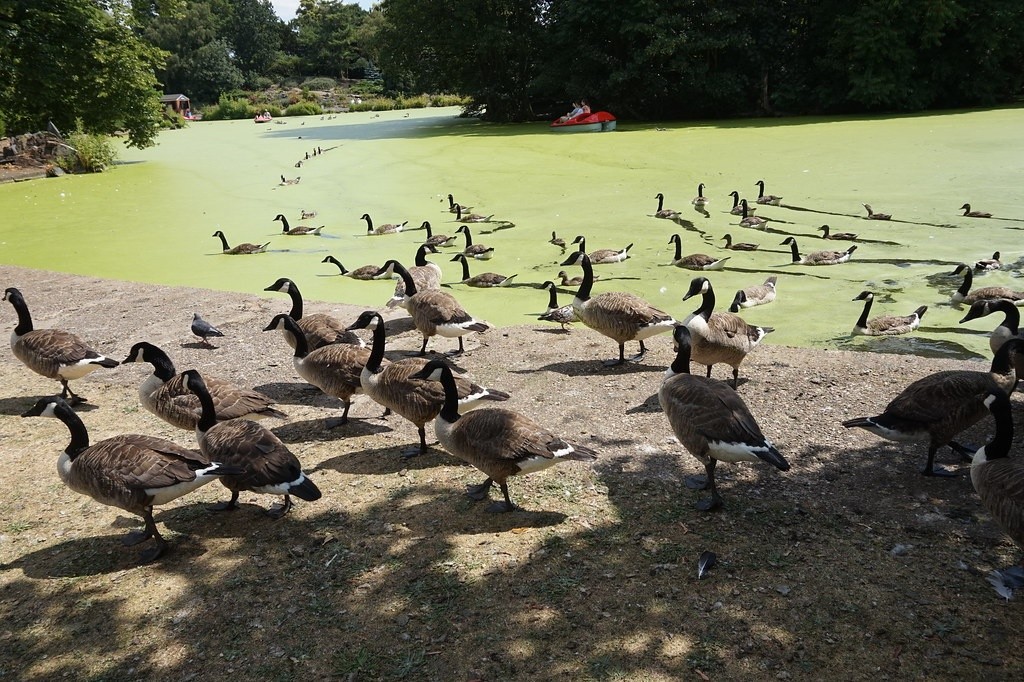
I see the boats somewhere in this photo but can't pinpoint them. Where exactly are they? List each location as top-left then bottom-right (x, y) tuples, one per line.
(549, 110), (617, 132)
(182, 115), (197, 121)
(254, 115), (273, 123)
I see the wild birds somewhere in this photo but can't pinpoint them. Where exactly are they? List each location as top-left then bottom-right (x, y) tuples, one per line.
(5, 194), (681, 555)
(186, 313), (226, 343)
(655, 180), (1024, 587)
(295, 146), (323, 168)
(278, 175), (301, 187)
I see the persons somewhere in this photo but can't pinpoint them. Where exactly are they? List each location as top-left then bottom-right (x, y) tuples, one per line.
(567, 98), (590, 118)
(263, 109), (270, 118)
(185, 109), (191, 117)
